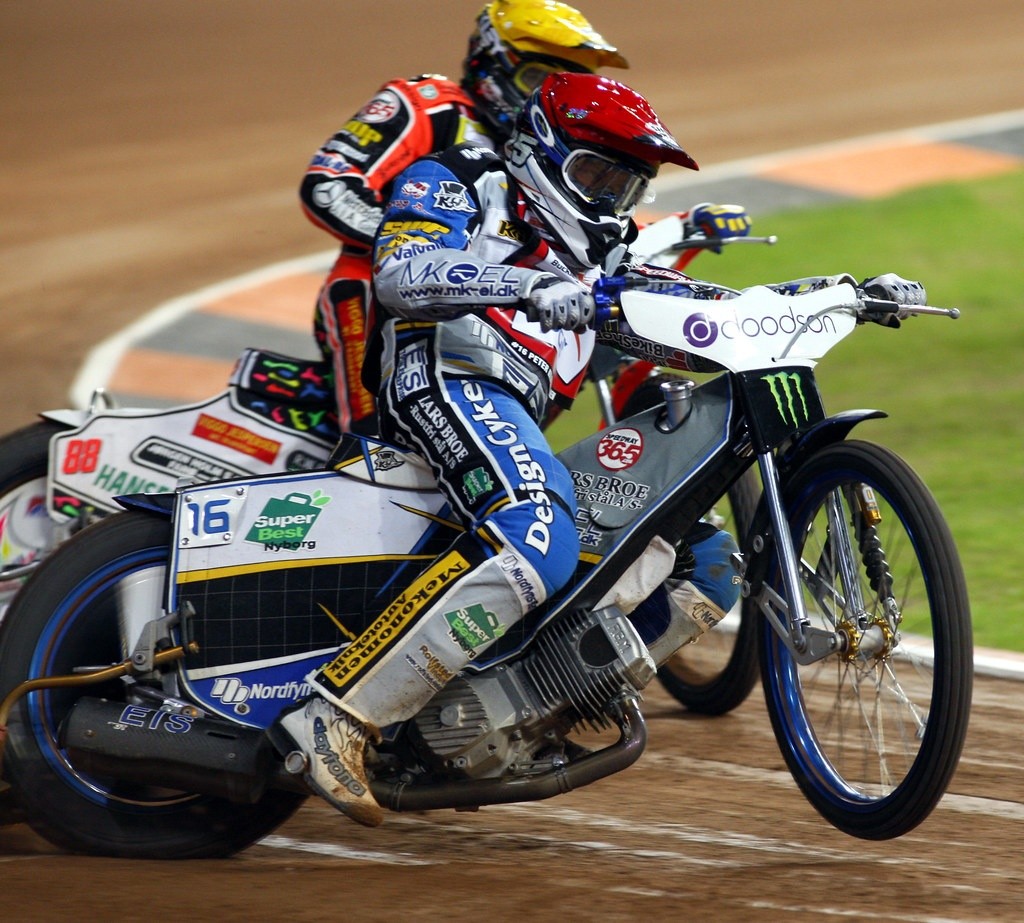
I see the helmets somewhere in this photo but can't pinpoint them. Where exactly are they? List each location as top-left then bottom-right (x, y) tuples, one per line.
(503, 71), (700, 267)
(461, 0), (617, 125)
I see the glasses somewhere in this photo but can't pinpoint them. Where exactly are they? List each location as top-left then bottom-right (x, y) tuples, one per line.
(496, 49), (568, 97)
(561, 148), (650, 219)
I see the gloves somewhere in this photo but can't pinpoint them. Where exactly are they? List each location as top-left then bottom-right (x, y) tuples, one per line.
(855, 272), (927, 328)
(688, 203), (753, 255)
(529, 276), (595, 334)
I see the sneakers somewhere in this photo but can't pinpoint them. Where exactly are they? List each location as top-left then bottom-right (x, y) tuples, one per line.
(263, 690), (385, 827)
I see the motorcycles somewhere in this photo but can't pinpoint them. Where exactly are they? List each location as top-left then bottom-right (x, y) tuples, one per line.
(0, 261), (978, 860)
(1, 208), (776, 624)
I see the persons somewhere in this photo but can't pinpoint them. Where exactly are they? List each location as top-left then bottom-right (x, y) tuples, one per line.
(259, 73), (923, 827)
(297, 0), (753, 472)
